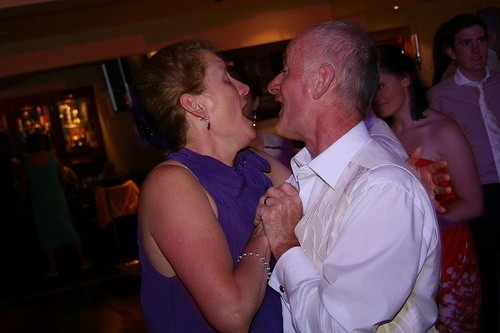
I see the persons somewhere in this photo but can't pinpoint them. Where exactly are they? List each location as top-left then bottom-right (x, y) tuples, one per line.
(128, 39), (454, 333)
(15, 131), (95, 280)
(234, 72), (291, 167)
(440, 7), (500, 81)
(426, 12), (500, 333)
(247, 19), (442, 332)
(371, 44), (485, 333)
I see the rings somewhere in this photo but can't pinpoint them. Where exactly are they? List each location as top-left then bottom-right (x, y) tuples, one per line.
(433, 185), (439, 195)
(263, 197), (271, 206)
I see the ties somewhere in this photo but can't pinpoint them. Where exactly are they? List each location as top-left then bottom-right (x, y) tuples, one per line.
(467, 79), (500, 176)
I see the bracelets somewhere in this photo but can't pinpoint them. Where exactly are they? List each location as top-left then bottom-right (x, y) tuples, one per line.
(236, 251), (272, 280)
